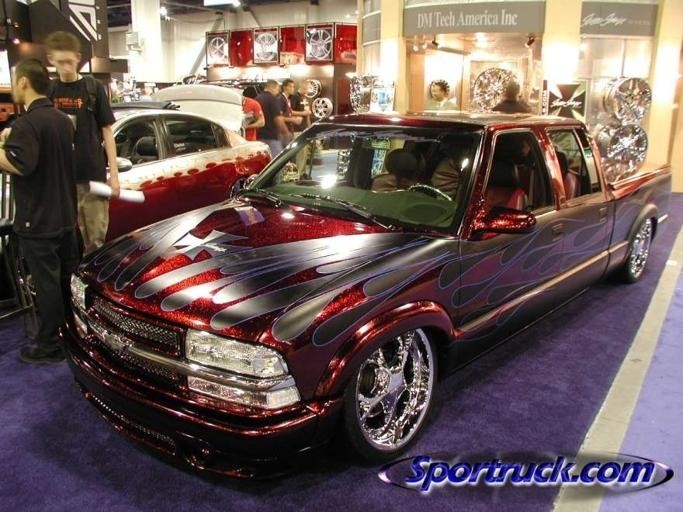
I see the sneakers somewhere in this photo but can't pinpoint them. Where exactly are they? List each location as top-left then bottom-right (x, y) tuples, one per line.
(20, 344), (64, 363)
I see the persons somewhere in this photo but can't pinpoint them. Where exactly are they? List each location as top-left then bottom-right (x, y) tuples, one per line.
(492, 82), (529, 113)
(1, 58), (80, 363)
(237, 80), (311, 184)
(425, 82), (459, 110)
(0, 31), (120, 277)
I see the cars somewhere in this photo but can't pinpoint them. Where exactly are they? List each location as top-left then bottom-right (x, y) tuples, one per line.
(0, 86), (20, 120)
(0, 84), (273, 312)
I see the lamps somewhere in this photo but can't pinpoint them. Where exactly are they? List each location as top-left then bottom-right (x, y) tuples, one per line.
(432, 34), (440, 48)
(525, 36), (535, 47)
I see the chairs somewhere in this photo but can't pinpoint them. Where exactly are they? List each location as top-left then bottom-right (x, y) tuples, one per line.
(486, 159), (529, 210)
(520, 151), (576, 201)
(131, 136), (157, 163)
(115, 135), (130, 157)
(184, 130), (209, 151)
(371, 148), (424, 191)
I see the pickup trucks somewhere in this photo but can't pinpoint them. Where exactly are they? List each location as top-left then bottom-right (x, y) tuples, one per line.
(61, 105), (673, 490)
